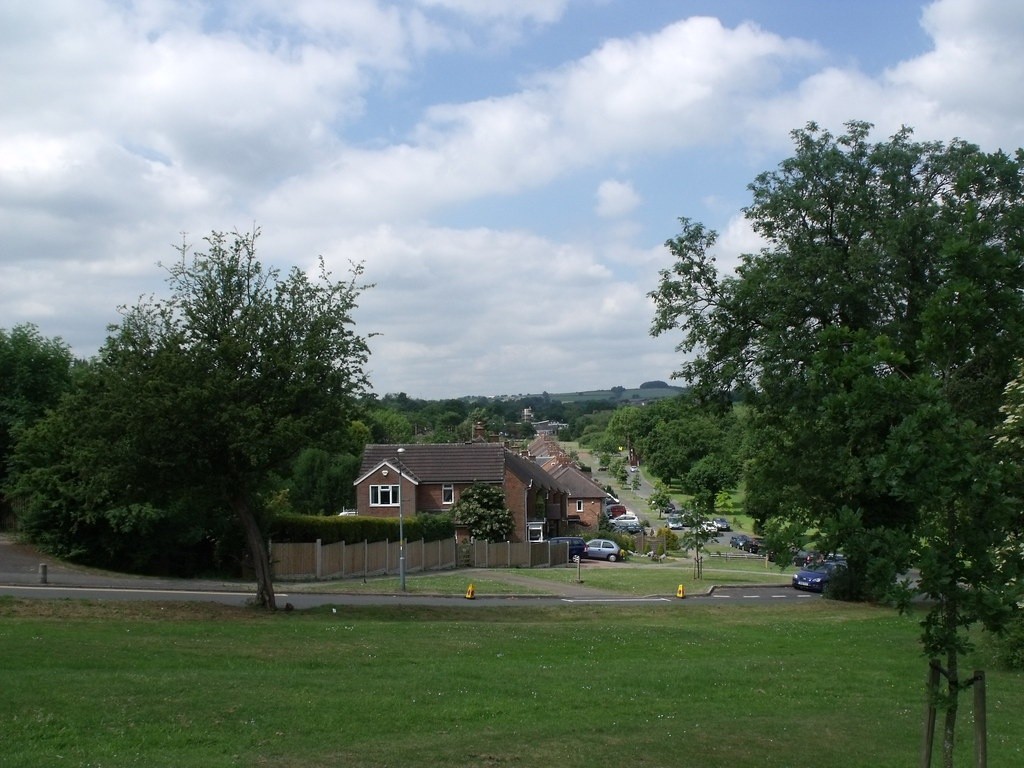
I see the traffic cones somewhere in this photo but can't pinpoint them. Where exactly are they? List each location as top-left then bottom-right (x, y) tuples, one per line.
(464, 583), (476, 600)
(676, 585), (685, 598)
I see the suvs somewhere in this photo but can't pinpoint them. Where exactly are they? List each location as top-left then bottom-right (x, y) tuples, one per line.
(545, 535), (589, 563)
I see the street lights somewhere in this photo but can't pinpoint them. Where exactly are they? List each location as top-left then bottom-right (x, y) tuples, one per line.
(396, 447), (406, 591)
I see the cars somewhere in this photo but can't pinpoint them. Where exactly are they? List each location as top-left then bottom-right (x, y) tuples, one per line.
(586, 539), (622, 562)
(792, 556), (848, 593)
(630, 465), (638, 472)
(605, 491), (843, 567)
